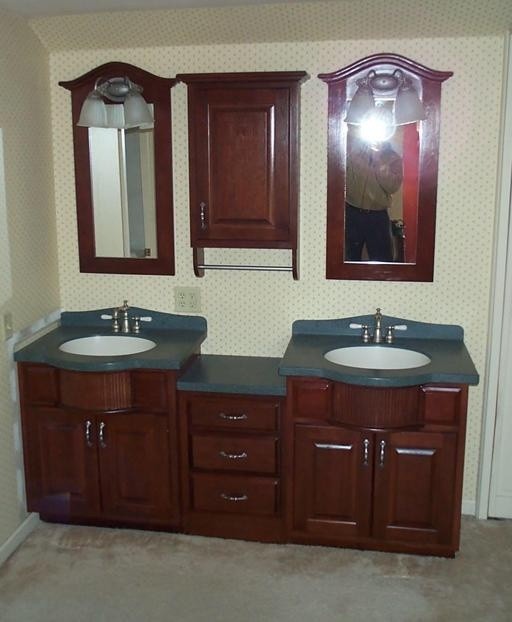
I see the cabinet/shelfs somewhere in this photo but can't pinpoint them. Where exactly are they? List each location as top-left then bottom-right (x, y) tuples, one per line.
(285, 375), (467, 558)
(178, 391), (285, 544)
(18, 364), (179, 533)
(178, 72), (310, 281)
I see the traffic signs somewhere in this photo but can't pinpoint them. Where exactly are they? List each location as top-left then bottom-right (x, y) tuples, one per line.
(174, 286), (200, 313)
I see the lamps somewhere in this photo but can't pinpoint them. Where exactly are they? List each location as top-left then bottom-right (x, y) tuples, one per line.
(76, 76), (153, 129)
(346, 69), (428, 128)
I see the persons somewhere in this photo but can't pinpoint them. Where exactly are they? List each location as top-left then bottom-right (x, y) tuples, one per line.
(345, 112), (403, 264)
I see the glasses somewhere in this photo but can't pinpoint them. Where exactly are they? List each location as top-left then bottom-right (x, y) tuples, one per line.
(346, 69), (428, 128)
(76, 76), (153, 129)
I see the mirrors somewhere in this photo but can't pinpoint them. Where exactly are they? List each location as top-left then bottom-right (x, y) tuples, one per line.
(58, 61), (175, 276)
(318, 52), (453, 283)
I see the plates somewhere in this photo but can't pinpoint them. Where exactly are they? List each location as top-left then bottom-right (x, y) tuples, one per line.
(178, 72), (310, 281)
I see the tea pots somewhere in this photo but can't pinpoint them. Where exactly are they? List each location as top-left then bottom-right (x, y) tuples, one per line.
(318, 52), (453, 283)
(58, 61), (175, 276)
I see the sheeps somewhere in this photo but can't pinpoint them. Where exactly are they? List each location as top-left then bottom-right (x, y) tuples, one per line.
(114, 298), (130, 334)
(372, 308), (384, 344)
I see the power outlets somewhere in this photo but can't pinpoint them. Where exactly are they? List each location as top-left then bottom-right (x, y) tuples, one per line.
(174, 286), (200, 313)
